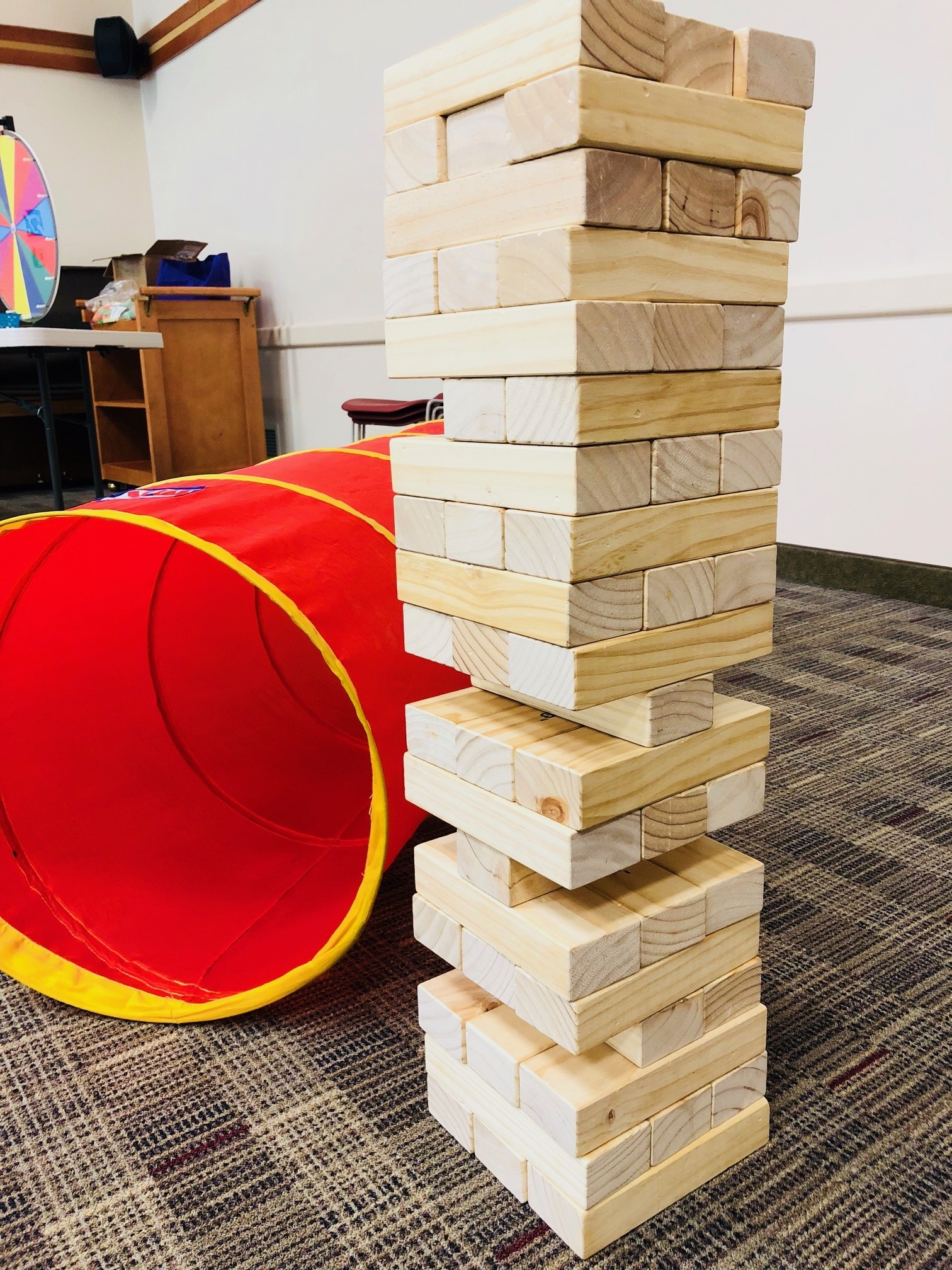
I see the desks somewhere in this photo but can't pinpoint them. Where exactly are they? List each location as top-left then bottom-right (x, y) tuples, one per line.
(0, 326), (164, 511)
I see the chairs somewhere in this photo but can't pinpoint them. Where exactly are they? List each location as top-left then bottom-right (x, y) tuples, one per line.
(341, 393), (445, 442)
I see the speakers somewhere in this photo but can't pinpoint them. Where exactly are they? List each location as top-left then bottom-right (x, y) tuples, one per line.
(93, 16), (150, 79)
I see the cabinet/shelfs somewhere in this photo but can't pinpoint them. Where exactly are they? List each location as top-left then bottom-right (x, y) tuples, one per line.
(75, 288), (266, 485)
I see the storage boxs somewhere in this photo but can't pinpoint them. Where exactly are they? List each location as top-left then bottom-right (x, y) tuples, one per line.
(91, 239), (207, 290)
